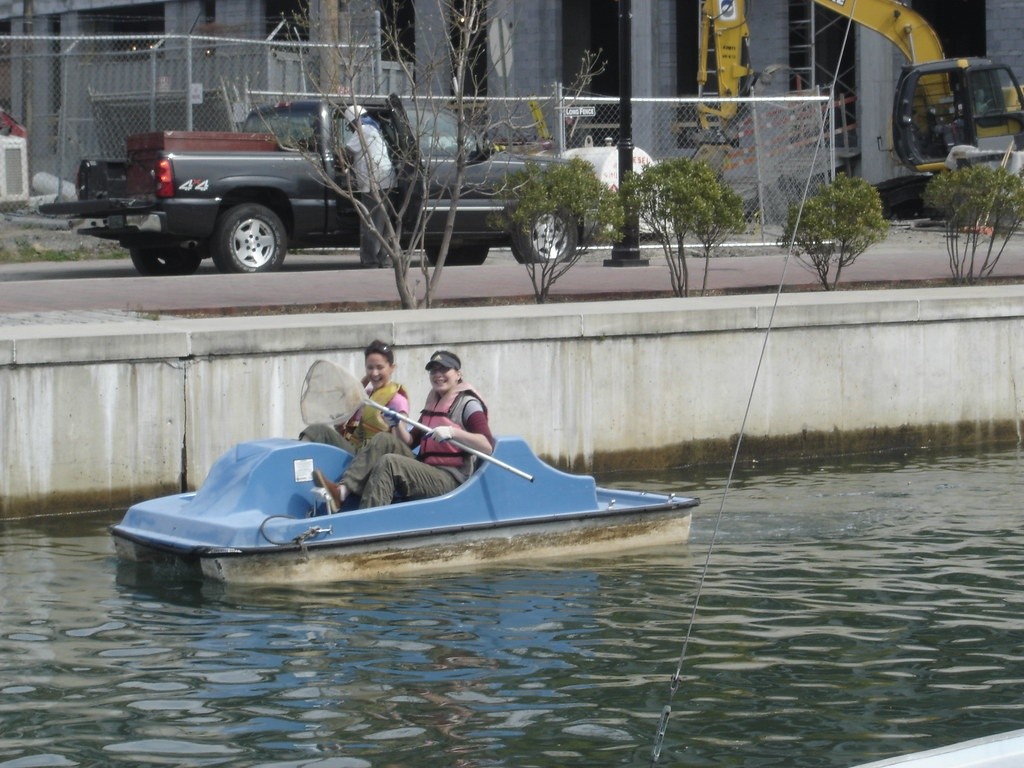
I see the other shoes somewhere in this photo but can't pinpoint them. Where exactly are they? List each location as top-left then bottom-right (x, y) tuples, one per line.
(361, 256), (395, 269)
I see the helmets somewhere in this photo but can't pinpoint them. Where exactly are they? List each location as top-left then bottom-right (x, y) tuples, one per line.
(344, 103), (368, 125)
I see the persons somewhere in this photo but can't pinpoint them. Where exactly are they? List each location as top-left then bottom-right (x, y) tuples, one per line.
(299, 340), (409, 456)
(344, 104), (398, 268)
(313, 351), (494, 508)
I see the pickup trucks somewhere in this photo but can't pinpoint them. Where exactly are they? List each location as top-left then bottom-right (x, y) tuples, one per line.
(37, 92), (598, 276)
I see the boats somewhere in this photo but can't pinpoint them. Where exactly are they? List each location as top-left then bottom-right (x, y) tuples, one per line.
(107, 435), (700, 587)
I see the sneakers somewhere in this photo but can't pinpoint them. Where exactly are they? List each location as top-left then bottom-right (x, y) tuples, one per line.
(312, 469), (345, 513)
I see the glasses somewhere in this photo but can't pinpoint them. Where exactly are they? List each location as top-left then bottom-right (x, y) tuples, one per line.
(427, 368), (453, 373)
(366, 344), (392, 354)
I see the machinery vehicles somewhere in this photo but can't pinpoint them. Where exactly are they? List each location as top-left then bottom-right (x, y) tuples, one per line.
(692, 1), (1024, 216)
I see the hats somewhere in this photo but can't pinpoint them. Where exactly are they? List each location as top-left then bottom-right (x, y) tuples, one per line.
(425, 353), (461, 370)
